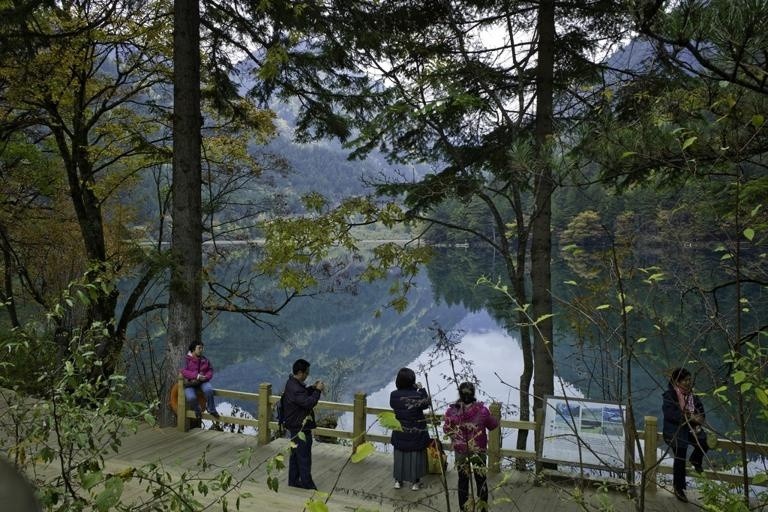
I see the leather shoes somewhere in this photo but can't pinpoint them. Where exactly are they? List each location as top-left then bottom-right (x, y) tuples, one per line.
(690, 459), (703, 473)
(674, 487), (687, 502)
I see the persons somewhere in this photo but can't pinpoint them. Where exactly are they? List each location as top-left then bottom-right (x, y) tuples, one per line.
(662, 368), (708, 503)
(282, 359), (325, 491)
(390, 367), (431, 491)
(443, 382), (498, 512)
(181, 340), (219, 420)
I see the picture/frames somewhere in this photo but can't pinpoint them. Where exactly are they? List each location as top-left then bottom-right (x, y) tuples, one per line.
(537, 394), (628, 473)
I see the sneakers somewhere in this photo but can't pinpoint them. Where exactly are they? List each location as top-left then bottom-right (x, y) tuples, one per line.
(393, 479), (403, 488)
(210, 412), (219, 418)
(196, 413), (201, 418)
(413, 481), (424, 490)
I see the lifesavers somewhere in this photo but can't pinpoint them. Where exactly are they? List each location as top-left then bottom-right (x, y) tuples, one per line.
(171, 382), (206, 416)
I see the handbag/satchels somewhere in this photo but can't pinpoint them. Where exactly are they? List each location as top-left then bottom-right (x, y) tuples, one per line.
(703, 426), (718, 450)
(273, 399), (283, 423)
(183, 377), (202, 387)
